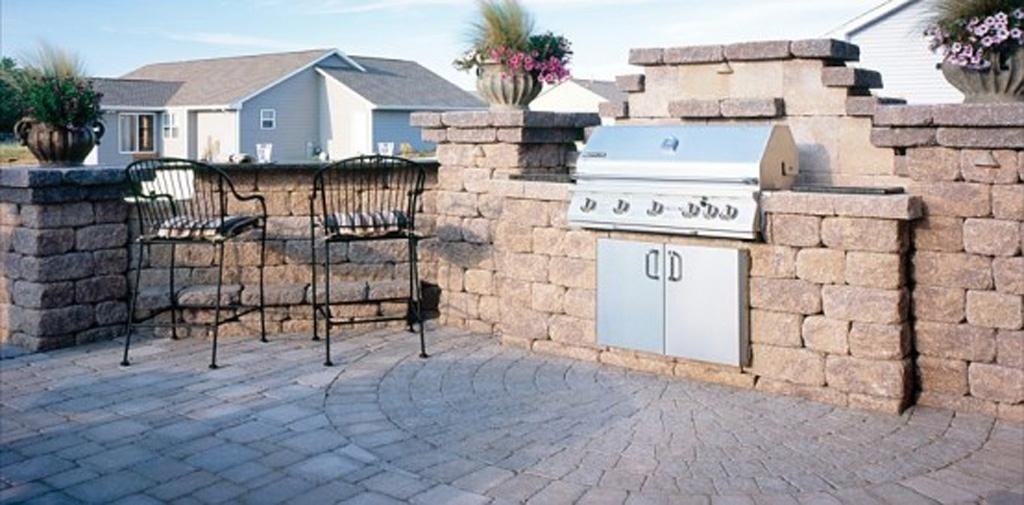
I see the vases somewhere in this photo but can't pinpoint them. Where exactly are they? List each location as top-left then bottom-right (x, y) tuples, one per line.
(476, 60), (542, 110)
(940, 47), (1024, 103)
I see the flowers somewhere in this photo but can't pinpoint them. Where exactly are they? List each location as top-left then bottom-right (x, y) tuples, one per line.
(451, 0), (574, 82)
(915, 0), (1024, 71)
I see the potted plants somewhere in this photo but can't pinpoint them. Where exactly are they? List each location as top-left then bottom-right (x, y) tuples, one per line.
(0, 42), (105, 166)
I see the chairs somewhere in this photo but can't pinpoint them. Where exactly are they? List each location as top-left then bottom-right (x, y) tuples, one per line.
(308, 151), (430, 367)
(120, 152), (268, 369)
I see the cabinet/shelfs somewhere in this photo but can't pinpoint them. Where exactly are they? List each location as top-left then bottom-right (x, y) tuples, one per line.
(596, 239), (749, 368)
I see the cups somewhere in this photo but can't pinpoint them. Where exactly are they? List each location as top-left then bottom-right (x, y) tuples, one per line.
(206, 134), (221, 164)
(255, 143), (271, 164)
(379, 142), (395, 163)
